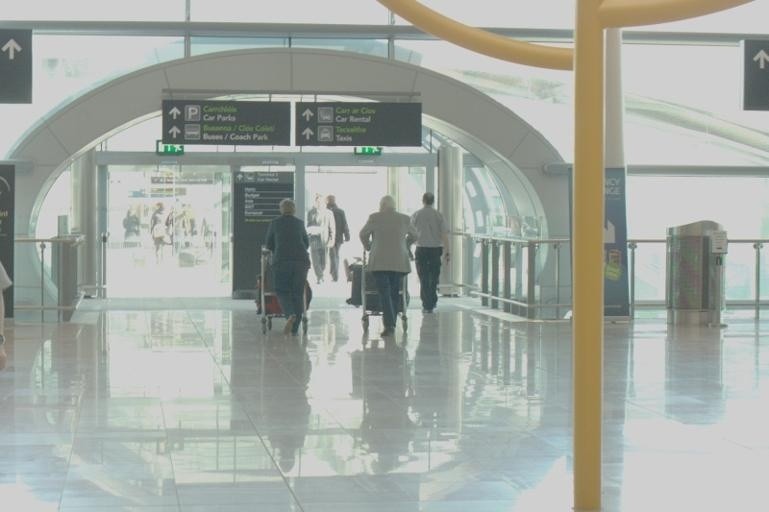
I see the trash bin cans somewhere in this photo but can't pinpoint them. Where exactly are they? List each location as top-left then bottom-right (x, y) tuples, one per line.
(667, 221), (727, 325)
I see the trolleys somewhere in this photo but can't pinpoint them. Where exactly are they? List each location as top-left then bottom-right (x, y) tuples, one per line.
(253, 244), (312, 336)
(357, 246), (411, 334)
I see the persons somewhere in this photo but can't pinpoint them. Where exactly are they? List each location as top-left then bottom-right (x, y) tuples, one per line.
(261, 199), (313, 336)
(408, 191), (452, 315)
(359, 194), (420, 339)
(116, 197), (215, 262)
(325, 194), (352, 283)
(305, 196), (336, 285)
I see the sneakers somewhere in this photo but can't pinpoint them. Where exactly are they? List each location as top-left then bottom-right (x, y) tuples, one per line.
(282, 312), (302, 336)
(380, 326), (396, 337)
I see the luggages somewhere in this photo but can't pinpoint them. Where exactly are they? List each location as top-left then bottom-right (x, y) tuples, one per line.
(255, 274), (312, 314)
(346, 263), (410, 312)
